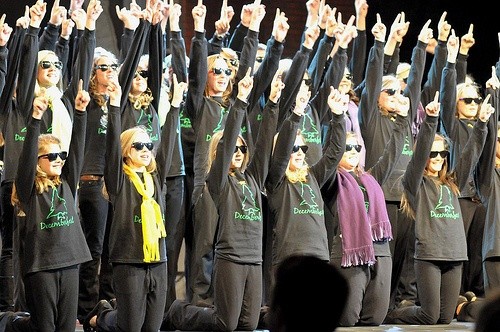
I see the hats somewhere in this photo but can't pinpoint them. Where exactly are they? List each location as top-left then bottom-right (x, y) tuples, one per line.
(37, 49), (56, 63)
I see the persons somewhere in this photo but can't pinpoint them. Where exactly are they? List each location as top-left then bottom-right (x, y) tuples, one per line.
(269, 252), (351, 332)
(0, 1), (500, 332)
(473, 289), (500, 332)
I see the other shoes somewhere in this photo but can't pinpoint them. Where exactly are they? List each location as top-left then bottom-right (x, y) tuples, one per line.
(161, 300), (192, 332)
(0, 311), (30, 332)
(384, 298), (415, 323)
(83, 298), (117, 332)
(455, 292), (476, 318)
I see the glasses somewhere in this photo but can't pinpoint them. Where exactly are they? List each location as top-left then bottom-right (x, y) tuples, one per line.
(210, 67), (232, 76)
(224, 58), (238, 67)
(458, 97), (481, 105)
(133, 70), (148, 79)
(292, 144), (309, 154)
(95, 64), (118, 71)
(381, 88), (403, 95)
(345, 73), (351, 81)
(402, 77), (407, 84)
(429, 151), (447, 158)
(38, 151), (67, 162)
(39, 61), (63, 69)
(234, 146), (247, 154)
(302, 78), (313, 86)
(132, 142), (154, 151)
(345, 144), (362, 152)
(256, 57), (263, 63)
